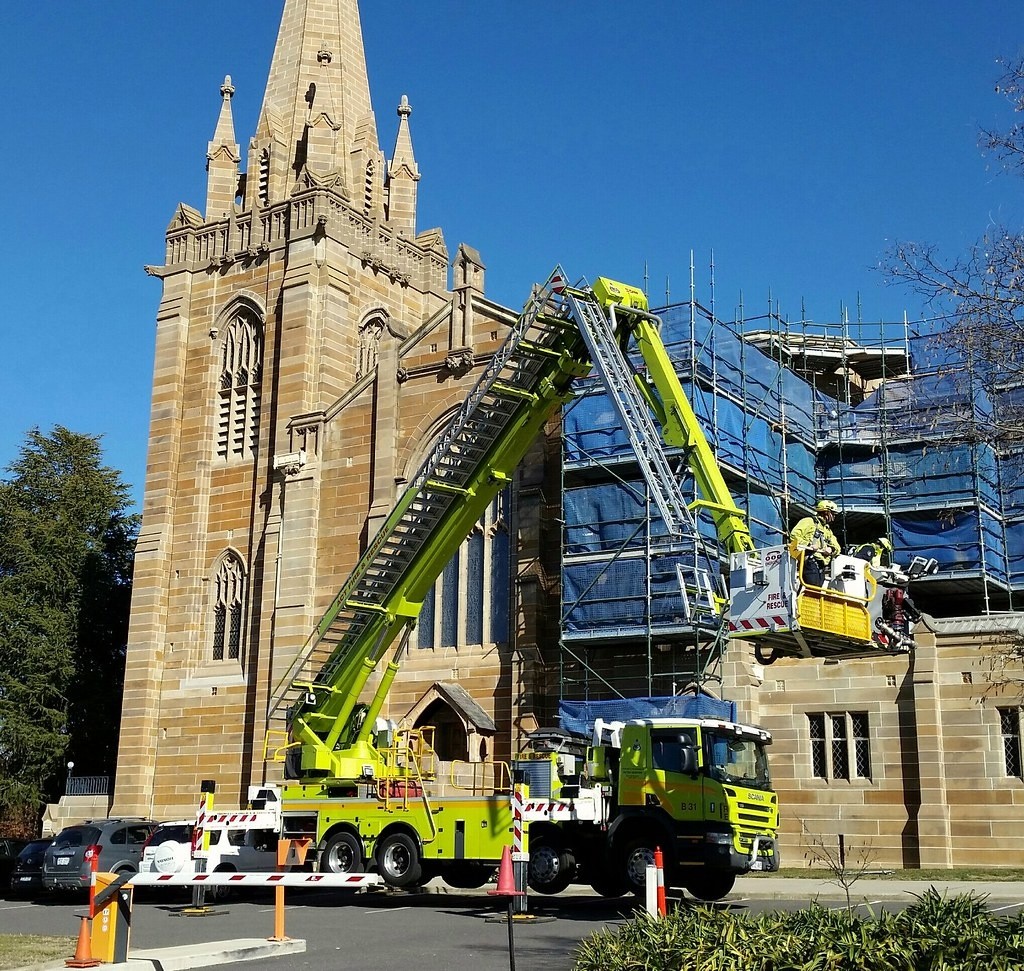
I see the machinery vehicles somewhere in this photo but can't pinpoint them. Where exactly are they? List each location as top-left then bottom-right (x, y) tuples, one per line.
(180, 264), (942, 924)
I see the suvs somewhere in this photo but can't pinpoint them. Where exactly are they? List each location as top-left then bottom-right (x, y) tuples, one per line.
(137, 817), (297, 905)
(42, 817), (160, 904)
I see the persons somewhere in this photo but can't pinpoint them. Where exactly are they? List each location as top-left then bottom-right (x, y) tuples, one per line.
(854, 537), (894, 567)
(790, 500), (841, 599)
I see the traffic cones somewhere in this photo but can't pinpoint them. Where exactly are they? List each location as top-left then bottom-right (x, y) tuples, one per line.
(64, 917), (102, 967)
(487, 844), (526, 896)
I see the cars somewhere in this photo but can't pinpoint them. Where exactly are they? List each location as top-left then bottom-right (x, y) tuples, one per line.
(9, 837), (54, 899)
(0, 838), (31, 900)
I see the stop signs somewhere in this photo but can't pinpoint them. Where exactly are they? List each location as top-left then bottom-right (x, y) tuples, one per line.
(551, 275), (566, 294)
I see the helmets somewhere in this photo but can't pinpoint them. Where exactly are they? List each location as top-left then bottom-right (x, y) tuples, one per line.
(816, 500), (837, 512)
(879, 538), (892, 550)
